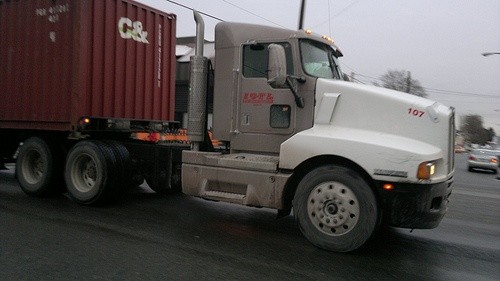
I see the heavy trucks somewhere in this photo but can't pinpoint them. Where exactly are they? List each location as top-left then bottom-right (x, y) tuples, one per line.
(0, 0), (456, 253)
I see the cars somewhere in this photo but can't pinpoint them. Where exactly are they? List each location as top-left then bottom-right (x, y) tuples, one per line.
(468, 143), (500, 174)
